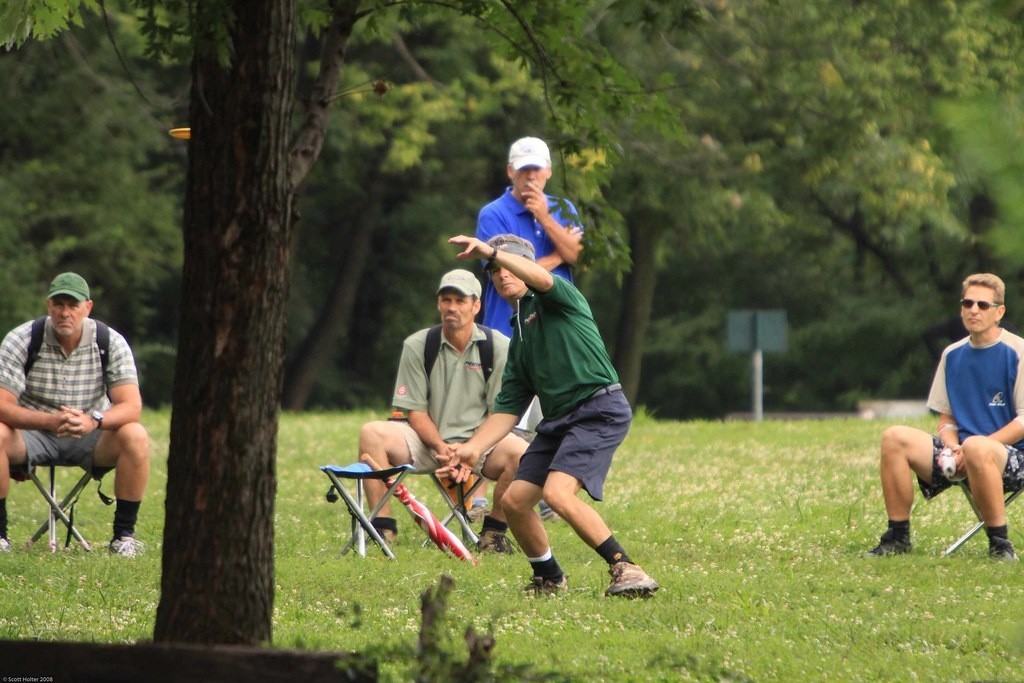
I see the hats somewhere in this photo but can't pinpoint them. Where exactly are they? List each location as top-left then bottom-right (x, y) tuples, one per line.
(481, 233), (535, 272)
(508, 136), (552, 170)
(435, 268), (482, 301)
(48, 271), (90, 301)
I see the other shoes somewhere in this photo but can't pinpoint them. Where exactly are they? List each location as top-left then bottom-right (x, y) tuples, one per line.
(539, 500), (560, 523)
(466, 504), (490, 522)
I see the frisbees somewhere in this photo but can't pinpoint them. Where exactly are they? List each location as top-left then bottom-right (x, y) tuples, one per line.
(168, 127), (191, 139)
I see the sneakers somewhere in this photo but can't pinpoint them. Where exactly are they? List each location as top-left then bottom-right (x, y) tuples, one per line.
(861, 527), (911, 559)
(0, 535), (11, 554)
(477, 527), (522, 556)
(521, 576), (570, 600)
(989, 536), (1015, 563)
(110, 532), (144, 558)
(365, 527), (397, 546)
(604, 562), (660, 596)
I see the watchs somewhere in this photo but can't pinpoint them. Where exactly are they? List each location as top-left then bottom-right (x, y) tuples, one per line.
(92, 409), (103, 430)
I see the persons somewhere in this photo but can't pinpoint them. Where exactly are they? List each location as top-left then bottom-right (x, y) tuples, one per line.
(448, 235), (659, 602)
(358, 269), (531, 556)
(862, 273), (1024, 567)
(478, 135), (584, 520)
(0, 273), (150, 560)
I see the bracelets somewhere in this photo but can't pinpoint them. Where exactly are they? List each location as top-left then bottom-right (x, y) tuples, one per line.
(938, 423), (959, 435)
(488, 249), (497, 262)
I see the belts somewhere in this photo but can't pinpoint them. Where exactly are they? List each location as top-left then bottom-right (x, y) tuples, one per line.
(593, 383), (622, 398)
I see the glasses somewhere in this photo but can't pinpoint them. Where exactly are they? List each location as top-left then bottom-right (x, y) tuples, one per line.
(959, 298), (1001, 311)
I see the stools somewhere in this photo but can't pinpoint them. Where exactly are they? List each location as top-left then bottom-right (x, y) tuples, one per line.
(940, 480), (1024, 554)
(320, 462), (415, 561)
(22, 460), (92, 553)
(421, 473), (483, 551)
